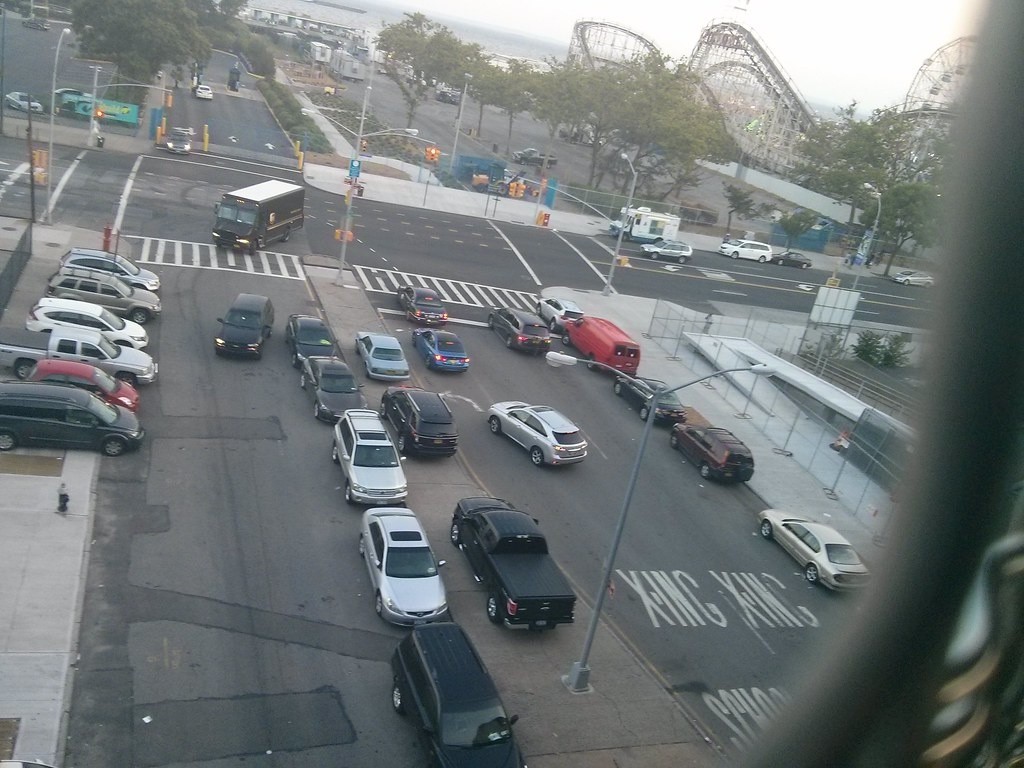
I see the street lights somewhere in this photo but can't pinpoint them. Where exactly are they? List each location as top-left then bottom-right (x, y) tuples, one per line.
(300, 86), (418, 284)
(46, 29), (71, 226)
(546, 351), (776, 693)
(601, 153), (638, 296)
(851, 182), (881, 289)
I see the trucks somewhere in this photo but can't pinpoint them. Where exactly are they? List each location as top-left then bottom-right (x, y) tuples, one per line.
(609, 205), (692, 265)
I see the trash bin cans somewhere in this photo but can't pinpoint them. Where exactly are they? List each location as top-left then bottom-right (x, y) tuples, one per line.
(97, 136), (105, 147)
(492, 144), (498, 153)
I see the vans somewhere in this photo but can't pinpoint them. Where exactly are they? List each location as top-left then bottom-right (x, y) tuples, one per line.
(717, 239), (811, 268)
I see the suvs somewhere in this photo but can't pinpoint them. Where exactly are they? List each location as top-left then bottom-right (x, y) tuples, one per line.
(489, 297), (755, 484)
(391, 622), (529, 768)
(0, 248), (162, 457)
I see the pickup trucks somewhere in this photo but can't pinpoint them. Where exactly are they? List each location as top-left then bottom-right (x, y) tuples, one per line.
(512, 148), (557, 169)
(451, 497), (577, 632)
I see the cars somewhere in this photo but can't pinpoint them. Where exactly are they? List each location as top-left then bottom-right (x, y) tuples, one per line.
(358, 507), (449, 627)
(6, 85), (96, 114)
(213, 285), (470, 507)
(166, 130), (193, 156)
(757, 509), (874, 593)
(192, 85), (213, 100)
(891, 270), (935, 289)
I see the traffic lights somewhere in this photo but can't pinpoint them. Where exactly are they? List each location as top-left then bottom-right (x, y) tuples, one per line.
(535, 211), (550, 228)
(361, 140), (367, 153)
(509, 182), (526, 199)
(424, 147), (441, 162)
(32, 150), (46, 186)
(167, 95), (173, 107)
